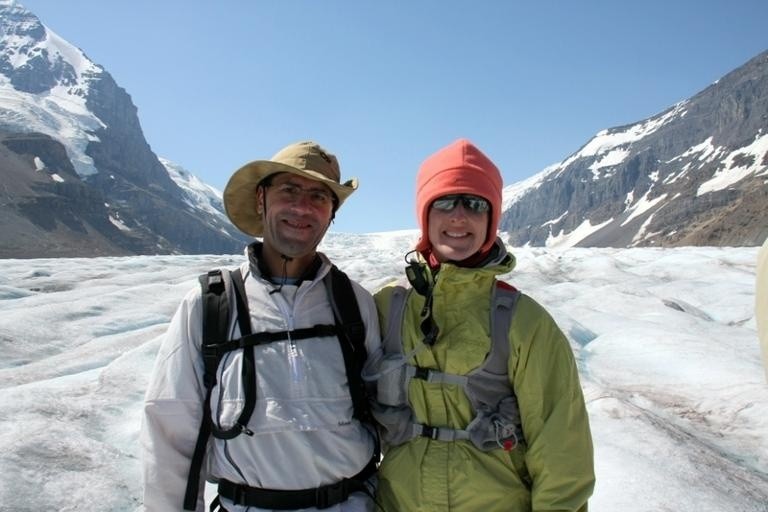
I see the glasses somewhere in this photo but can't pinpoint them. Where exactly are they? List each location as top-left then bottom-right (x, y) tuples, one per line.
(430, 192), (492, 214)
(260, 178), (337, 208)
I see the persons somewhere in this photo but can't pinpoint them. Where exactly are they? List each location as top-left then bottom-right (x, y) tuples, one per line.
(369, 136), (597, 512)
(138, 141), (385, 511)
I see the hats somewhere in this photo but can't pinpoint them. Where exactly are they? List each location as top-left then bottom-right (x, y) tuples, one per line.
(413, 138), (504, 257)
(223, 139), (359, 238)
